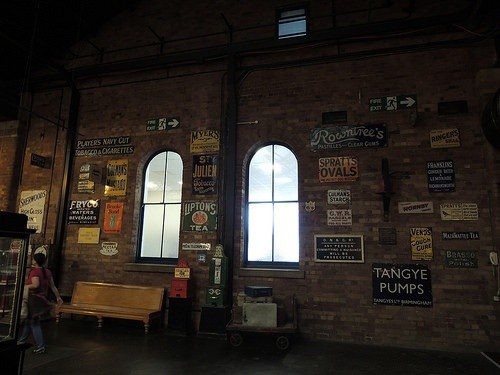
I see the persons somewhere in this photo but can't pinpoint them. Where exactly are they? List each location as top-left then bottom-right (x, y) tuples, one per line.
(16, 253), (63, 352)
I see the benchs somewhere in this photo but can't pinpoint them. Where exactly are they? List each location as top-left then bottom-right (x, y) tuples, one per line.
(54, 281), (165, 340)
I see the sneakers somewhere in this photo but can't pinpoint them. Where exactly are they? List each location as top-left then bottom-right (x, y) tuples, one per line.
(15, 337), (29, 344)
(33, 346), (46, 353)
